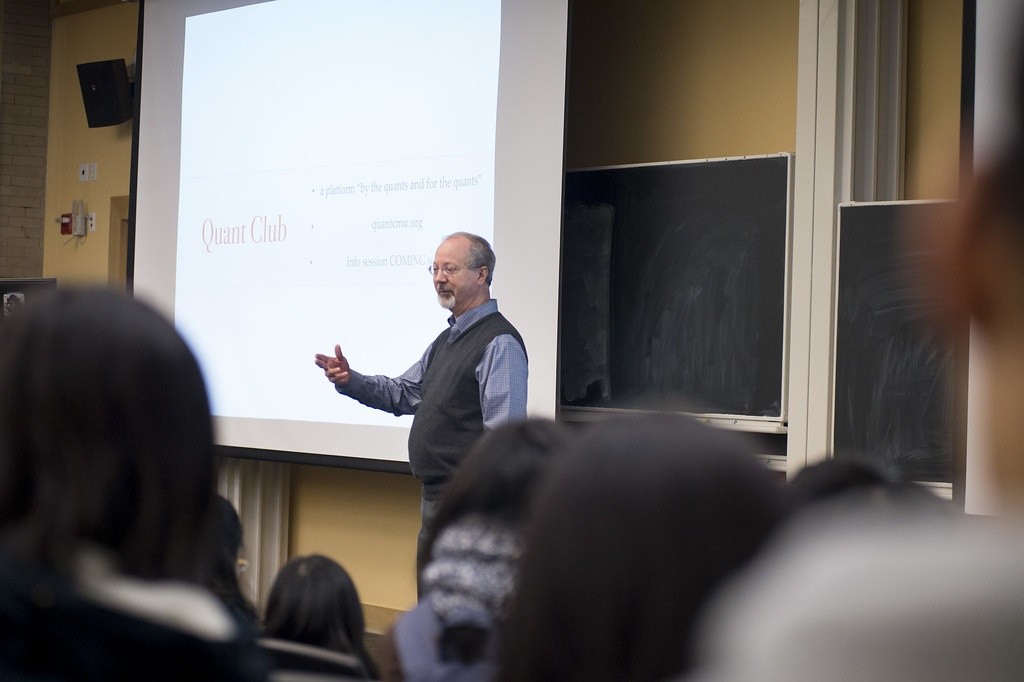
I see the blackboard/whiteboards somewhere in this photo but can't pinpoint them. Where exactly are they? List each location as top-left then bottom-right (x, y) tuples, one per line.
(829, 198), (959, 503)
(556, 154), (796, 433)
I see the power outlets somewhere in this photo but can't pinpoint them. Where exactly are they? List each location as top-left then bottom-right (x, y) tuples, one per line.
(88, 212), (95, 232)
(79, 163), (89, 182)
(89, 163), (97, 180)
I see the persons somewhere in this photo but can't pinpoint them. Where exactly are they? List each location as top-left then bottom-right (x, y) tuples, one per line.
(315, 233), (528, 608)
(0, 141), (1024, 682)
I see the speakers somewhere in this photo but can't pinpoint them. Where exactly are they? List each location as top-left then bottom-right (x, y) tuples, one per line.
(76, 58), (134, 129)
